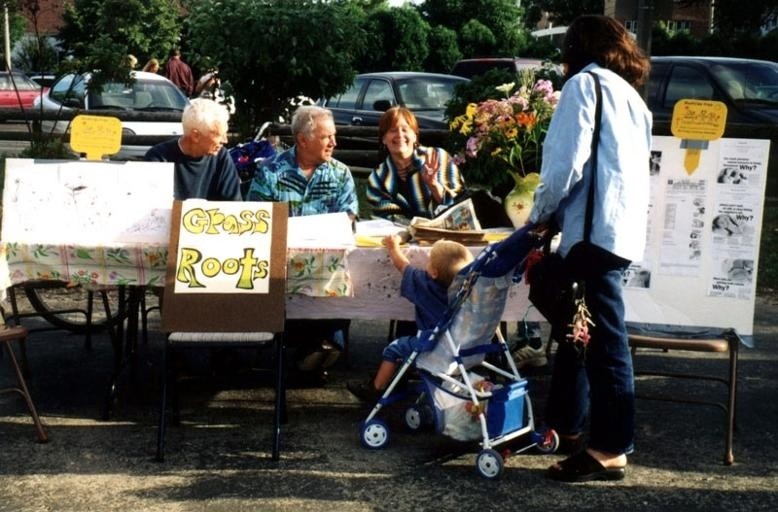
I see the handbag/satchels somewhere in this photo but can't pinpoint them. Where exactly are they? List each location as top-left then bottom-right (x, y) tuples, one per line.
(528, 253), (584, 325)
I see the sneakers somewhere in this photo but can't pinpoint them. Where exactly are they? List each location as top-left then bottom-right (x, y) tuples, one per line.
(346, 380), (385, 404)
(506, 344), (547, 369)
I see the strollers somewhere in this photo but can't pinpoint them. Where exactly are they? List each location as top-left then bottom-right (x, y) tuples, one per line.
(357, 222), (564, 479)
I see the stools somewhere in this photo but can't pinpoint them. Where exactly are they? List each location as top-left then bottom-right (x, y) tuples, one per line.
(625, 328), (745, 465)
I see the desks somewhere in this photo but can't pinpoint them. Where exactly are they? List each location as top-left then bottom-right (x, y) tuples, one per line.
(0, 226), (550, 429)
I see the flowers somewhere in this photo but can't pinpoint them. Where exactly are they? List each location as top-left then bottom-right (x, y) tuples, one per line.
(449, 68), (564, 177)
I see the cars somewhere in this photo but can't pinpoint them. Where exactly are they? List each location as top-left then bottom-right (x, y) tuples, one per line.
(29, 72), (56, 87)
(1, 67), (52, 123)
(274, 70), (487, 191)
(32, 70), (196, 163)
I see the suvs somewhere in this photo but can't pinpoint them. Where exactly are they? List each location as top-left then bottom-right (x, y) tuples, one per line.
(449, 52), (567, 94)
(638, 52), (777, 183)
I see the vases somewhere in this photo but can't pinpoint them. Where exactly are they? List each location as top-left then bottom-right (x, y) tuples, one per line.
(505, 170), (542, 232)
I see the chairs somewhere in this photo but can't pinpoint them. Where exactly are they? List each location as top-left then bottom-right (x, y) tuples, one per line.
(0, 255), (52, 445)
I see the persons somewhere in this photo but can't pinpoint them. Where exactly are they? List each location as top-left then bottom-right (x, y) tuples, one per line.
(245, 105), (360, 389)
(126, 54), (138, 69)
(141, 58), (160, 73)
(365, 106), (466, 226)
(164, 47), (194, 97)
(143, 98), (266, 378)
(513, 14), (654, 482)
(345, 234), (475, 412)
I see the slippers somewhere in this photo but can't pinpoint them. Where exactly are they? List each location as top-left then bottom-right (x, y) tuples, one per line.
(547, 447), (625, 481)
